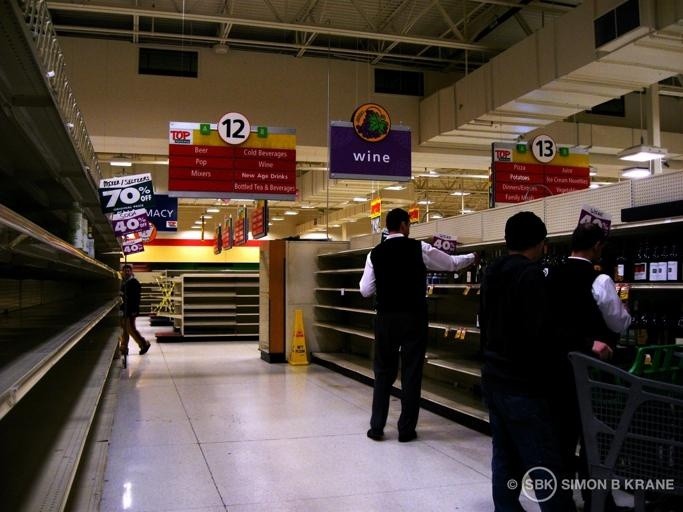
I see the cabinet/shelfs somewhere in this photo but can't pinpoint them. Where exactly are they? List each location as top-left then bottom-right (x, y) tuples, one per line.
(311, 213), (681, 463)
(138, 269), (258, 334)
(0, 1), (124, 512)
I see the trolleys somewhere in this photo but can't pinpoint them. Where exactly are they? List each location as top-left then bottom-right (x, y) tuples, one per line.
(565, 350), (682, 512)
(590, 343), (682, 467)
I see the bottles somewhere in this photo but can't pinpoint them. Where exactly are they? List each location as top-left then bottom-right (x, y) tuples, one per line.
(617, 296), (682, 348)
(67, 201), (95, 261)
(450, 247), (502, 285)
(540, 242), (682, 284)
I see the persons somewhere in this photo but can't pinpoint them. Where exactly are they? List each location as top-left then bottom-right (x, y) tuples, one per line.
(558, 221), (635, 511)
(483, 212), (616, 510)
(358, 207), (483, 442)
(120, 265), (151, 355)
